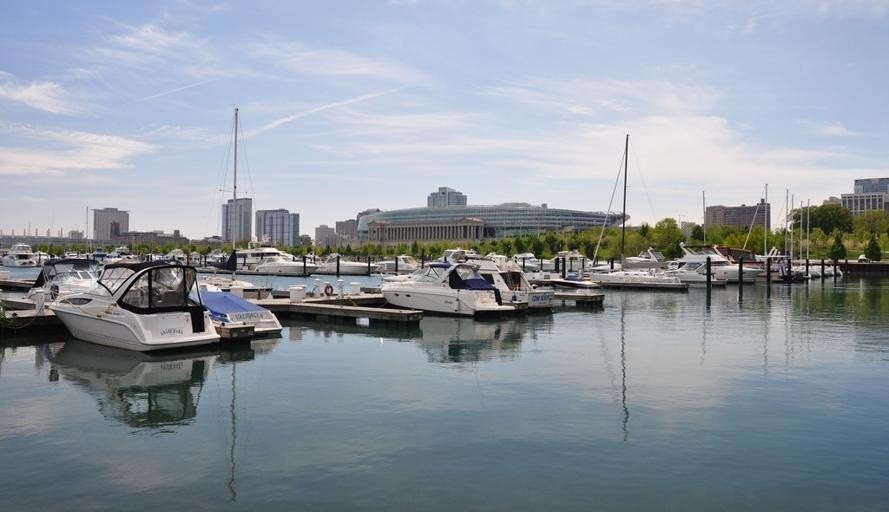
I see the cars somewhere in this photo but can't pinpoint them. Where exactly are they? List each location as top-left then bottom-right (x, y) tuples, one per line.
(857, 254), (871, 262)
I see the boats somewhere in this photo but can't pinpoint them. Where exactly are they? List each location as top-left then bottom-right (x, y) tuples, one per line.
(43, 339), (220, 437)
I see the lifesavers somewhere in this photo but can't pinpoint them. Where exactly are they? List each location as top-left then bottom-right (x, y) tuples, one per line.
(324, 285), (333, 295)
(50, 285), (59, 298)
